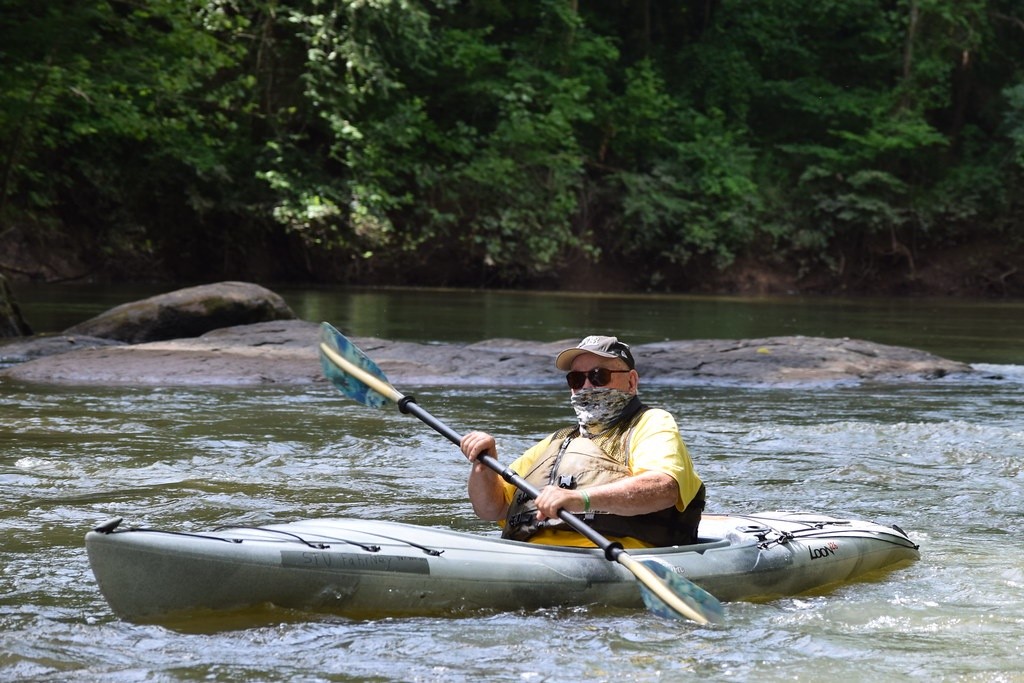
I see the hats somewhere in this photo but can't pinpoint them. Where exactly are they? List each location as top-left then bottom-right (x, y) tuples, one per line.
(555, 335), (634, 370)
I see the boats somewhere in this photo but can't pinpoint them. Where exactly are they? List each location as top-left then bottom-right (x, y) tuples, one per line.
(85, 512), (922, 636)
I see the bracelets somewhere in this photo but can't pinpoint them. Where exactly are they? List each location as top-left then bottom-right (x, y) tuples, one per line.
(581, 490), (589, 512)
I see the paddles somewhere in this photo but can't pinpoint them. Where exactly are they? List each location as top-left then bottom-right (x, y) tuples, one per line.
(315, 323), (726, 628)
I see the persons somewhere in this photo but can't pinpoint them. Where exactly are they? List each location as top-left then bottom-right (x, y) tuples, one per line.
(462, 336), (705, 556)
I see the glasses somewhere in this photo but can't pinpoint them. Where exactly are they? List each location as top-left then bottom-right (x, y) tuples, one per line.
(566, 367), (630, 390)
